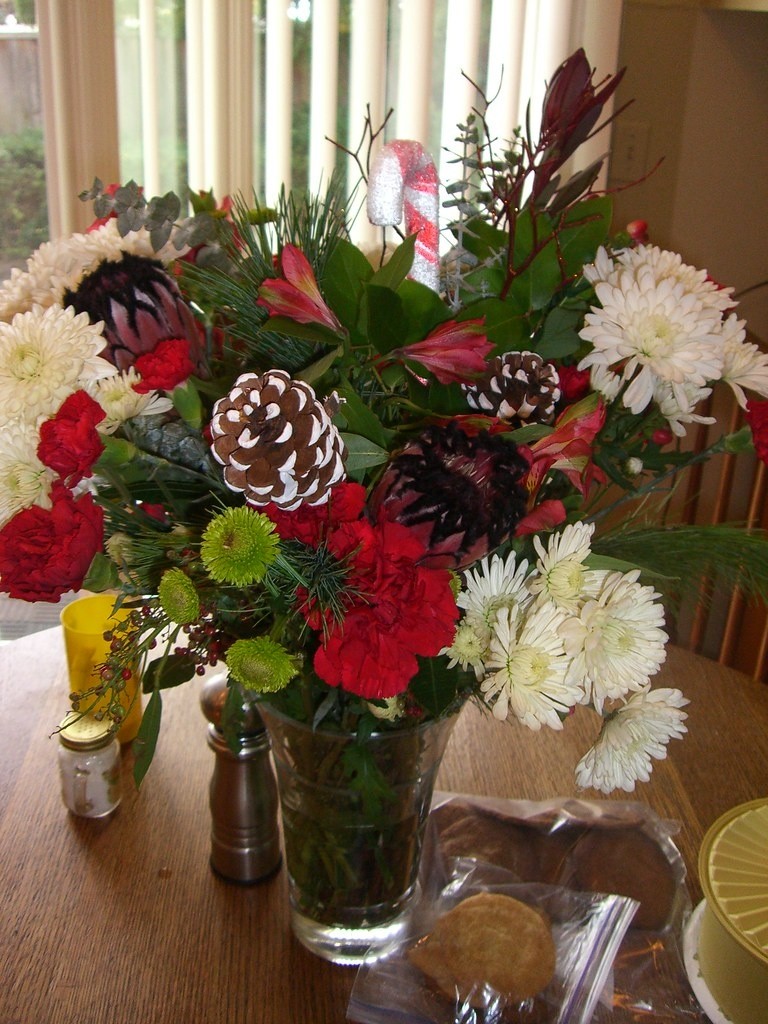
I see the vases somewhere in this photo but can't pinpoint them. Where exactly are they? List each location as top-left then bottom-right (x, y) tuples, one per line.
(248, 687), (472, 965)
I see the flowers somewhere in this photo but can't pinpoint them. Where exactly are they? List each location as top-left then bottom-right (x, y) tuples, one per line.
(0, 52), (768, 929)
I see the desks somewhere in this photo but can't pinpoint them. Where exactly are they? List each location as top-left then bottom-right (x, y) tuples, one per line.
(0, 604), (767, 1021)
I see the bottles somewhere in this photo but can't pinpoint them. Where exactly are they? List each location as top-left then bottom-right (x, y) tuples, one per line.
(59, 713), (125, 820)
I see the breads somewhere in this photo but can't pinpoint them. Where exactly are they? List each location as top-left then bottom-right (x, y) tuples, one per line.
(411, 893), (555, 1006)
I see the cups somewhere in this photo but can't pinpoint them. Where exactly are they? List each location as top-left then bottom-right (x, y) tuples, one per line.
(60, 594), (144, 745)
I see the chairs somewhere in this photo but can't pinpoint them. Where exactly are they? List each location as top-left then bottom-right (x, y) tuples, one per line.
(657, 276), (767, 685)
